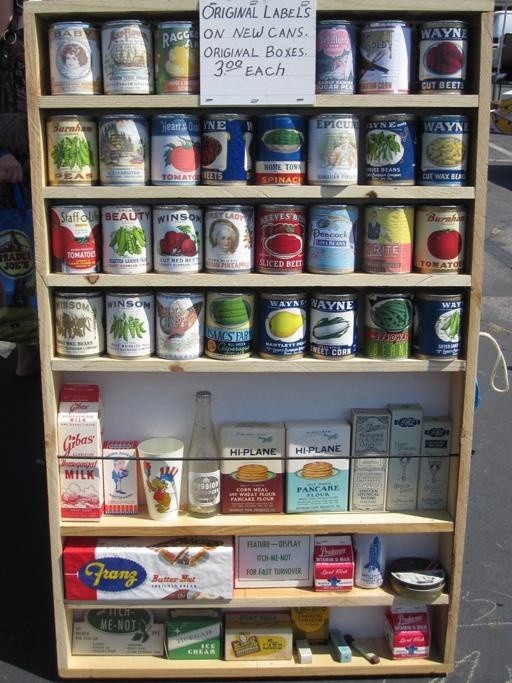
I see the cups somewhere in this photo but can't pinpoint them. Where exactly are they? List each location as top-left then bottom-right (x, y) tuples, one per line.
(136, 439), (184, 520)
(355, 534), (385, 587)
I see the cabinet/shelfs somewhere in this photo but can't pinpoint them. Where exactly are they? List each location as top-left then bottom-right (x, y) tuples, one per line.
(21, 0), (496, 679)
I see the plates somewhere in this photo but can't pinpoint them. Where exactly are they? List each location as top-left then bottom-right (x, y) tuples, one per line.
(390, 558), (446, 601)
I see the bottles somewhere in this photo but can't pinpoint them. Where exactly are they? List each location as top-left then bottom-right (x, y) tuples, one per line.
(185, 392), (222, 514)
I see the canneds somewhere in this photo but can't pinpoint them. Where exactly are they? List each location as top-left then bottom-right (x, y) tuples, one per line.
(363, 288), (414, 361)
(151, 204), (204, 275)
(46, 21), (103, 94)
(307, 203), (360, 275)
(155, 21), (201, 95)
(204, 204), (257, 275)
(420, 113), (471, 186)
(156, 288), (206, 359)
(47, 206), (102, 274)
(414, 287), (466, 362)
(358, 20), (412, 94)
(414, 19), (469, 93)
(44, 114), (99, 185)
(414, 204), (467, 274)
(255, 114), (310, 186)
(309, 287), (360, 360)
(258, 288), (309, 360)
(206, 288), (255, 361)
(363, 113), (417, 187)
(101, 205), (153, 275)
(149, 111), (204, 186)
(362, 203), (415, 275)
(254, 205), (308, 275)
(309, 113), (359, 185)
(105, 289), (155, 360)
(101, 19), (155, 96)
(316, 19), (360, 95)
(202, 111), (256, 186)
(53, 289), (106, 357)
(96, 112), (151, 185)
